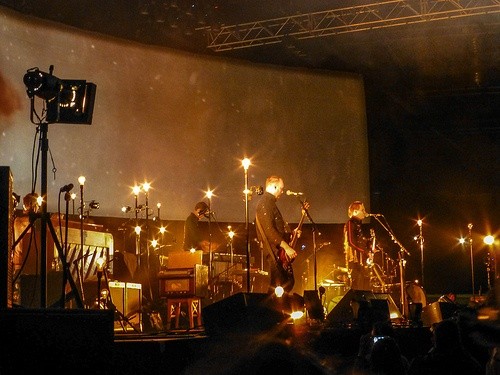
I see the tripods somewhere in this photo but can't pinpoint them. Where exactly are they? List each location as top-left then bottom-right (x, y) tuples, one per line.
(11, 123), (84, 309)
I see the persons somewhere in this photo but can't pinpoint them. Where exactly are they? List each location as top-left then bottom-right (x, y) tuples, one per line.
(345, 201), (375, 289)
(371, 323), (395, 349)
(408, 321), (485, 375)
(351, 335), (410, 375)
(14, 193), (41, 270)
(218, 342), (334, 375)
(183, 202), (209, 251)
(255, 175), (303, 296)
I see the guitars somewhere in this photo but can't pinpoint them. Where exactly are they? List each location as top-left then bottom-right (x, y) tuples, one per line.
(361, 228), (376, 277)
(277, 201), (311, 278)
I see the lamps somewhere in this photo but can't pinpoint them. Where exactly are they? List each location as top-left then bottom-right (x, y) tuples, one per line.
(23, 66), (76, 125)
(23, 193), (43, 213)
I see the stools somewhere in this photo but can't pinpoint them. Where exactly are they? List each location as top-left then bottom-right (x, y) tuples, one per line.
(168, 295), (202, 337)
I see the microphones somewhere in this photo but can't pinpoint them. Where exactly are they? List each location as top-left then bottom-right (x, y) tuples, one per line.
(365, 213), (382, 217)
(286, 189), (304, 196)
(59, 183), (73, 192)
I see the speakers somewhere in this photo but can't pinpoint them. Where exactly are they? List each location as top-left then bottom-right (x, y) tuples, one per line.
(328, 288), (390, 327)
(202, 292), (278, 335)
(47, 225), (143, 331)
(421, 302), (458, 327)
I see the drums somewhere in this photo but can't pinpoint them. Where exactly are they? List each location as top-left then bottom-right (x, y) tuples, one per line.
(388, 281), (427, 317)
